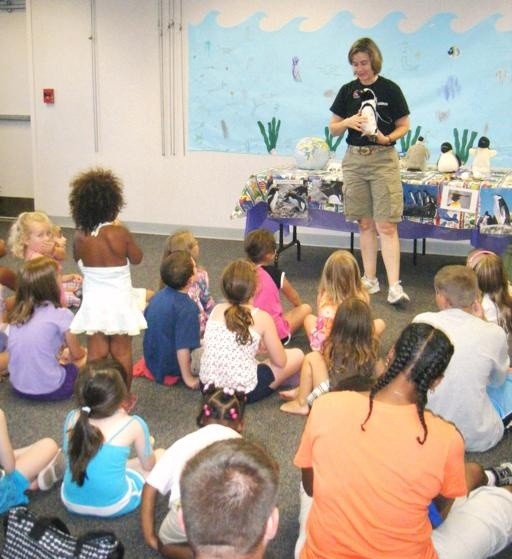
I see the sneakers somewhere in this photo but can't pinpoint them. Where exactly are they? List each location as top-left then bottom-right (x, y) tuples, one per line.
(488, 462), (512, 487)
(358, 274), (379, 294)
(387, 280), (411, 304)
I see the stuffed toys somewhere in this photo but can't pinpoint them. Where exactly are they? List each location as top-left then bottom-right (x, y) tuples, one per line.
(356, 87), (389, 140)
(436, 142), (462, 174)
(406, 136), (430, 172)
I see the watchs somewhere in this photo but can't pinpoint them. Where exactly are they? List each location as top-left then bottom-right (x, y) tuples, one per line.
(385, 133), (395, 147)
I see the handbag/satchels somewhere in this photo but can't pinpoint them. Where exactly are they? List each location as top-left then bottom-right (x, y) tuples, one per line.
(3, 506), (125, 559)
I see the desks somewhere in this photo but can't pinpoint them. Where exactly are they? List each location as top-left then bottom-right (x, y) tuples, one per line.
(230, 162), (512, 268)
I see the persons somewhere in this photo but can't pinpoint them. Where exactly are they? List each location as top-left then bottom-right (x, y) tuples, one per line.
(329, 36), (411, 306)
(409, 263), (512, 453)
(0, 235), (22, 389)
(293, 322), (512, 558)
(143, 250), (207, 391)
(6, 211), (82, 309)
(303, 249), (386, 355)
(140, 380), (248, 559)
(68, 168), (149, 414)
(468, 136), (498, 181)
(52, 224), (68, 252)
(293, 374), (512, 512)
(466, 248), (512, 365)
(197, 259), (304, 406)
(244, 229), (314, 347)
(0, 408), (68, 519)
(167, 231), (216, 339)
(59, 357), (167, 521)
(7, 256), (86, 404)
(279, 296), (384, 417)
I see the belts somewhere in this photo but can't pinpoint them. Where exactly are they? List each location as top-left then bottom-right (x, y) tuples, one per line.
(348, 145), (392, 154)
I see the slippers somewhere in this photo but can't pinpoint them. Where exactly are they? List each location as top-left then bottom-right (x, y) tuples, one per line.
(38, 448), (63, 492)
